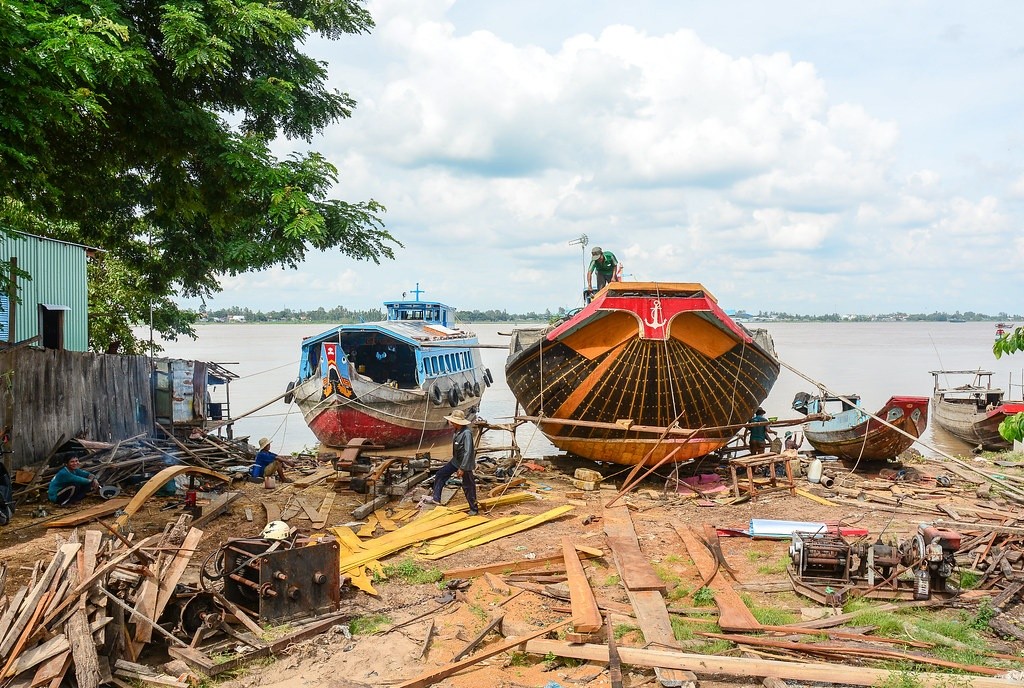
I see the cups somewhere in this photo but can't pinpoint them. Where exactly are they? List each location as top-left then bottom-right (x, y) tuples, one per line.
(249, 464), (261, 477)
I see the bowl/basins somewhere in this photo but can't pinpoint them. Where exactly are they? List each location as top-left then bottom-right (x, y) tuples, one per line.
(769, 417), (777, 422)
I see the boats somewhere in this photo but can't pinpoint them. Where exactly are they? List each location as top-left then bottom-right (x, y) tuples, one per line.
(803, 395), (930, 459)
(505, 281), (782, 467)
(928, 369), (1024, 452)
(291, 283), (487, 451)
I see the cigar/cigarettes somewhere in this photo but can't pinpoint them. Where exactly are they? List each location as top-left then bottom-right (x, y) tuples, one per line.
(99, 485), (102, 490)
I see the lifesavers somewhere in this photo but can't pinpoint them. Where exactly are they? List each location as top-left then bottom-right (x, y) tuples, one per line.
(285, 381), (295, 404)
(483, 375), (490, 387)
(429, 385), (444, 406)
(447, 382), (481, 407)
(486, 368), (494, 384)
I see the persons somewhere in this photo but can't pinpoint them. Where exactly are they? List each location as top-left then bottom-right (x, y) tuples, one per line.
(587, 246), (620, 300)
(47, 452), (102, 508)
(743, 407), (777, 456)
(428, 409), (478, 516)
(255, 438), (295, 483)
(784, 431), (804, 450)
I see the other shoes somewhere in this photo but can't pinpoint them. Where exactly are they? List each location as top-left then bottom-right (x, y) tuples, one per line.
(469, 510), (478, 516)
(279, 476), (295, 483)
(425, 499), (441, 505)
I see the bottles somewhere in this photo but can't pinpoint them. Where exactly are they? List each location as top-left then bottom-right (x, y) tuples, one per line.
(265, 476), (275, 489)
(807, 458), (822, 483)
(913, 559), (931, 600)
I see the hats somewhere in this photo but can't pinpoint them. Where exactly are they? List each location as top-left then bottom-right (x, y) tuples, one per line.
(785, 431), (792, 439)
(259, 438), (273, 452)
(443, 410), (472, 425)
(756, 407), (766, 415)
(591, 247), (601, 260)
